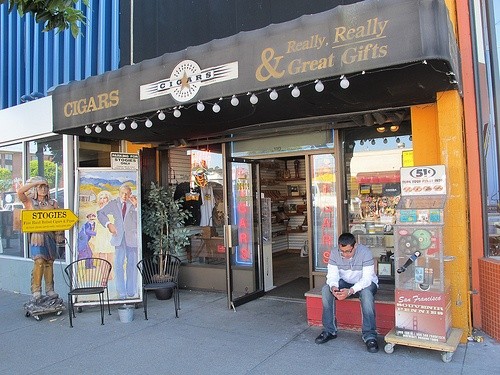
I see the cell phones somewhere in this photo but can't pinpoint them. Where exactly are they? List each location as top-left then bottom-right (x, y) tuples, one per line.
(335, 290), (341, 292)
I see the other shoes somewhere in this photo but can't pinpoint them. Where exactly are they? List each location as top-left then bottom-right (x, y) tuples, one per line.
(315, 330), (338, 344)
(366, 338), (378, 353)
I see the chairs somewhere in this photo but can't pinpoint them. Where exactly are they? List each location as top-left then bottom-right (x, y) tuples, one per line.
(136, 253), (182, 320)
(64, 257), (112, 328)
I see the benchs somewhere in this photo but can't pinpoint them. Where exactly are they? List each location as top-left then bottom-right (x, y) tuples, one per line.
(305, 284), (395, 335)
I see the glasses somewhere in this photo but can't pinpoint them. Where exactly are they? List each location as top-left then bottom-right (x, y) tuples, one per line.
(338, 247), (354, 254)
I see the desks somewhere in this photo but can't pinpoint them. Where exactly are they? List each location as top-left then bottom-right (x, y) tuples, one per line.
(185, 235), (226, 263)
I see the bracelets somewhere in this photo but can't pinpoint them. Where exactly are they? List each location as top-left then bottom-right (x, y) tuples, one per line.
(346, 289), (352, 296)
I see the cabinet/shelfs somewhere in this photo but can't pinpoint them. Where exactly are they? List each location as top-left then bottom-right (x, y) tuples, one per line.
(259, 168), (308, 238)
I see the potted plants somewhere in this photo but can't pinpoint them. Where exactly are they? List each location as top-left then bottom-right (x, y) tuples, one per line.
(141, 181), (204, 300)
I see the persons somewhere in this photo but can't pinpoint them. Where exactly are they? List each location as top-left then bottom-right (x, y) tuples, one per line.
(18, 178), (62, 300)
(315, 232), (379, 353)
